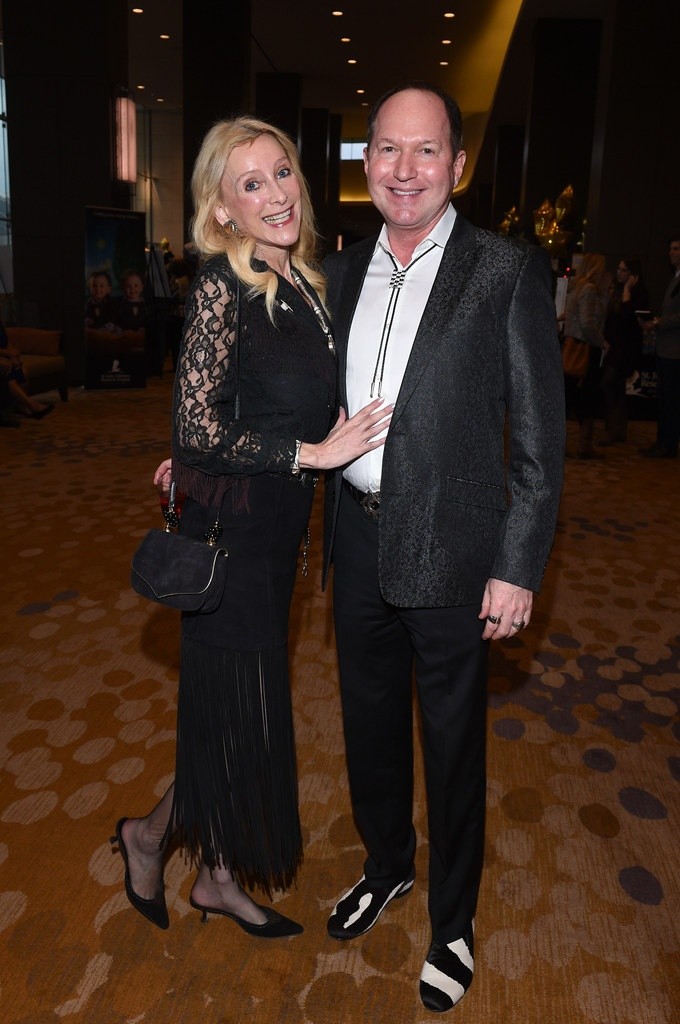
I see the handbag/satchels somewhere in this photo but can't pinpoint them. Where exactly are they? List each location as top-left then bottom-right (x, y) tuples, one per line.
(562, 336), (592, 373)
(131, 528), (234, 618)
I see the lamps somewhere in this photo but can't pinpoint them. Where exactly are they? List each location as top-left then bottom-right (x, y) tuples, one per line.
(115, 94), (137, 184)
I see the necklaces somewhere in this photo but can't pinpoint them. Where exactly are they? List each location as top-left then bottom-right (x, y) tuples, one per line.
(369, 244), (436, 397)
(274, 271), (336, 357)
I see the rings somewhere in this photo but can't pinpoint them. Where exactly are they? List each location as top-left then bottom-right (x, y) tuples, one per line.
(488, 615), (501, 624)
(512, 621), (525, 630)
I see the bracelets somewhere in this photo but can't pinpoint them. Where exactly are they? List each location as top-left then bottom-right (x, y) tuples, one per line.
(290, 438), (302, 475)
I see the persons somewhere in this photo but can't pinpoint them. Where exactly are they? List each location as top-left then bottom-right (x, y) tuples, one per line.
(152, 82), (567, 1014)
(558, 237), (680, 461)
(0, 320), (55, 420)
(85, 269), (151, 331)
(92, 118), (397, 938)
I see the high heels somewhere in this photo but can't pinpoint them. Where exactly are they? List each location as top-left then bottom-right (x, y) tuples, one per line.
(110, 817), (169, 930)
(189, 896), (306, 937)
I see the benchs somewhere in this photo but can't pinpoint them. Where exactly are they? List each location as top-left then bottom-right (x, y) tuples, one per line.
(5, 326), (68, 401)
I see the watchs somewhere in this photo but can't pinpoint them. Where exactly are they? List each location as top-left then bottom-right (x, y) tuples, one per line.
(653, 320), (658, 326)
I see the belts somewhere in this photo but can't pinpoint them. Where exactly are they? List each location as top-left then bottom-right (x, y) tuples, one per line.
(343, 480), (382, 519)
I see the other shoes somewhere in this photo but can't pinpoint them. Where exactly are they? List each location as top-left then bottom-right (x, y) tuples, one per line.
(644, 444), (677, 461)
(327, 865), (416, 940)
(420, 917), (476, 1012)
(34, 403), (55, 420)
(565, 450), (604, 460)
(601, 431), (631, 446)
